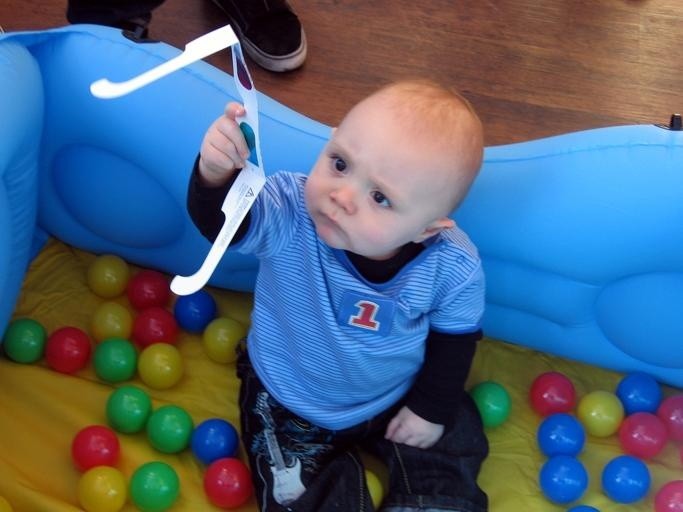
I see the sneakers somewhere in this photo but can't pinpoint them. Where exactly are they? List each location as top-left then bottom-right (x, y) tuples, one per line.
(205, 0), (310, 75)
(68, 0), (156, 42)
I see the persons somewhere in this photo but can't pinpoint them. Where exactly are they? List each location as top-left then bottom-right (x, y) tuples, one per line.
(66, 1), (308, 74)
(186, 74), (496, 511)
